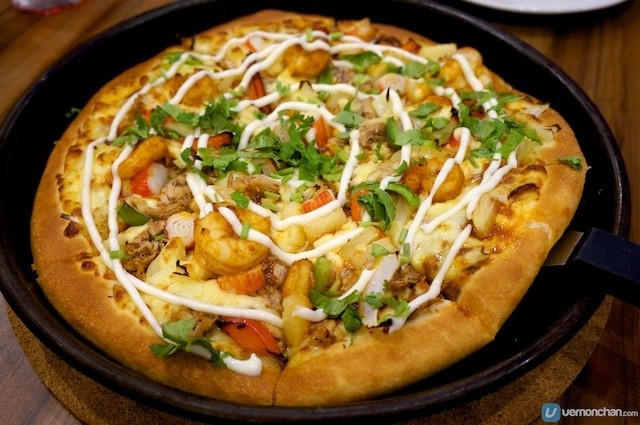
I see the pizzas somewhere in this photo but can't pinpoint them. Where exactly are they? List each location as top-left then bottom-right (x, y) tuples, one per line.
(27, 8), (590, 409)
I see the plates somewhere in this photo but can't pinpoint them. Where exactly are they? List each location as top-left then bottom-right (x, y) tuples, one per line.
(0, 0), (632, 425)
